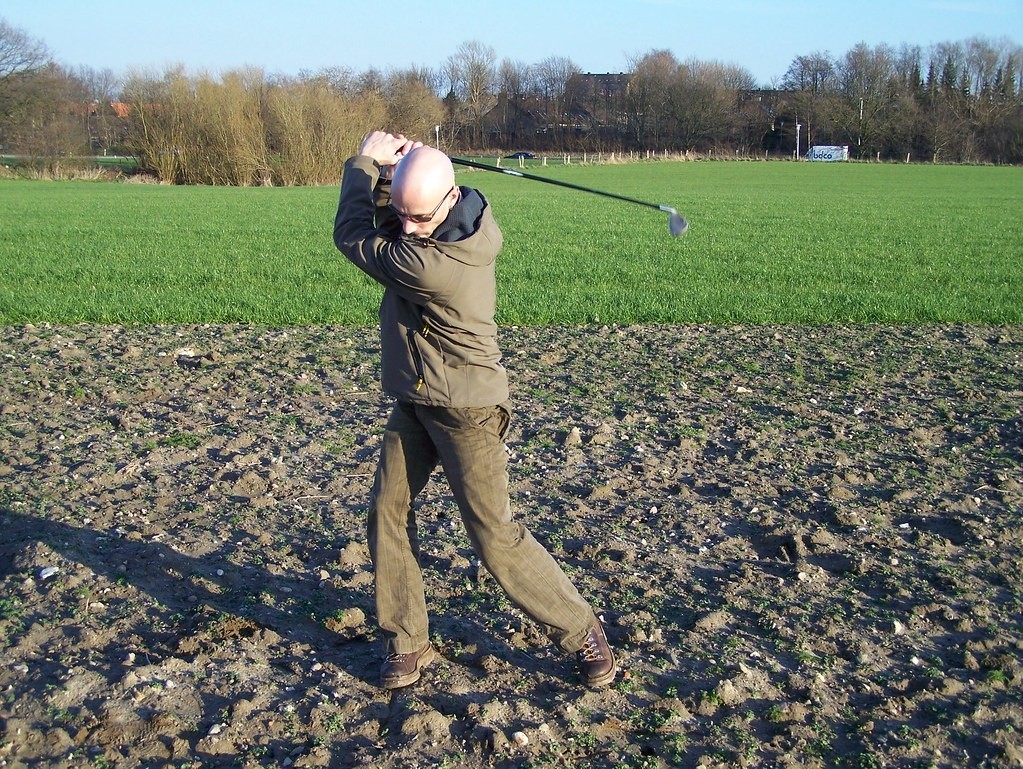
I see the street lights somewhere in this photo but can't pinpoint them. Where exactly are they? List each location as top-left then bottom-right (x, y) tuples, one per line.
(435, 125), (440, 150)
(796, 124), (802, 160)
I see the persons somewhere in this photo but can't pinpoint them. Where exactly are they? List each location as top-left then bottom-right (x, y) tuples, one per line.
(333, 131), (616, 688)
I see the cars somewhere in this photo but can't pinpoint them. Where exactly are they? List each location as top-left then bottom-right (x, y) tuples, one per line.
(504, 152), (537, 159)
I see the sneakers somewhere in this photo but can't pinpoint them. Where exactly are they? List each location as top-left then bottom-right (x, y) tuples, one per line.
(379, 640), (434, 688)
(574, 620), (617, 686)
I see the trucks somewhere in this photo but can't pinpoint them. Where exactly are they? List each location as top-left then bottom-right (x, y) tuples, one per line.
(804, 146), (848, 161)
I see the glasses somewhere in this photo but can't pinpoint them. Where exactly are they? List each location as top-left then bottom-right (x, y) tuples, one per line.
(386, 186), (454, 223)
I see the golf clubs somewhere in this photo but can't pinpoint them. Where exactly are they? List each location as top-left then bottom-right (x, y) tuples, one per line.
(396, 147), (689, 236)
